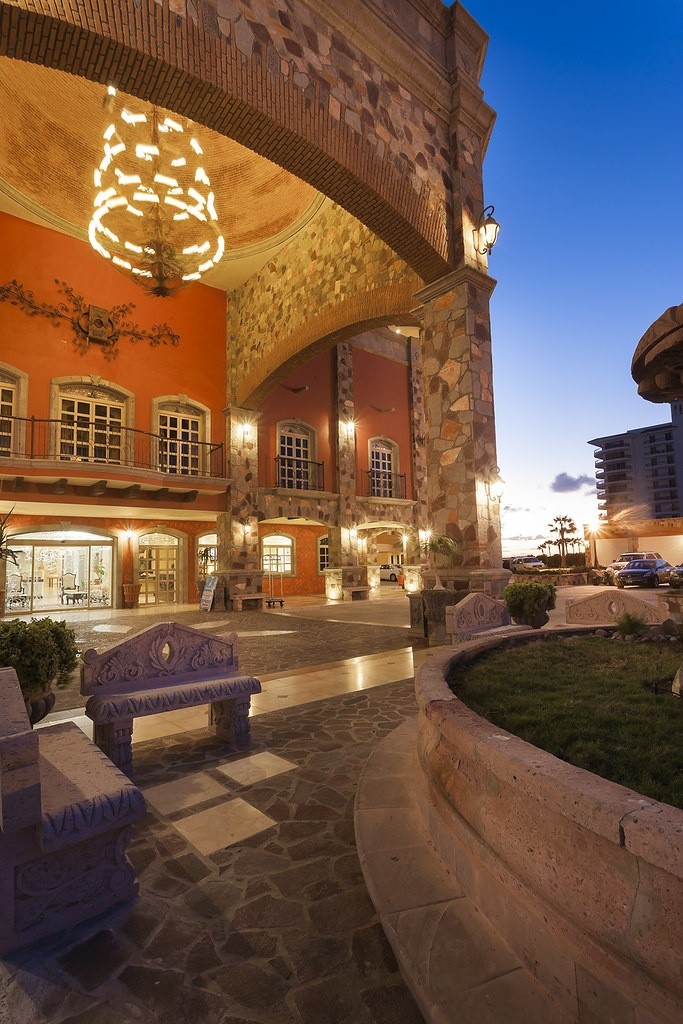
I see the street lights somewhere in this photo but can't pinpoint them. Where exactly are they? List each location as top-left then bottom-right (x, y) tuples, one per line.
(588, 523), (600, 570)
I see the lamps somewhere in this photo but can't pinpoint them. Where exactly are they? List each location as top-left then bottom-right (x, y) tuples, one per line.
(89, 85), (225, 298)
(472, 205), (500, 256)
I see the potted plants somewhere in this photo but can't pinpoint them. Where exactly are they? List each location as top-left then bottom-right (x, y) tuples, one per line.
(0, 615), (82, 729)
(413, 533), (459, 644)
(504, 580), (558, 629)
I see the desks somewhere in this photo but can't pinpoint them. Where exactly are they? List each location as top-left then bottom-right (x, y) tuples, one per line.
(65, 592), (87, 605)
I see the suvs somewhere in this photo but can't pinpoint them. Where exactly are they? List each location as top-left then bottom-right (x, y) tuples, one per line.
(606, 551), (664, 577)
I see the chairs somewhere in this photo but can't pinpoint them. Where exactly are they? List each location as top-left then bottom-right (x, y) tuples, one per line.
(7, 574), (27, 610)
(61, 573), (80, 605)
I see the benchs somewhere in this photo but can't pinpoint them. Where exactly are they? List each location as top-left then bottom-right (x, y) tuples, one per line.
(445, 592), (532, 646)
(556, 590), (672, 628)
(230, 593), (269, 612)
(80, 622), (262, 774)
(341, 585), (371, 601)
(0, 666), (147, 956)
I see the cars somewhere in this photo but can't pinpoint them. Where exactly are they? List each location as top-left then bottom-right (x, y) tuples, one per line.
(615, 559), (677, 588)
(378, 563), (404, 581)
(511, 557), (546, 572)
(667, 562), (683, 590)
